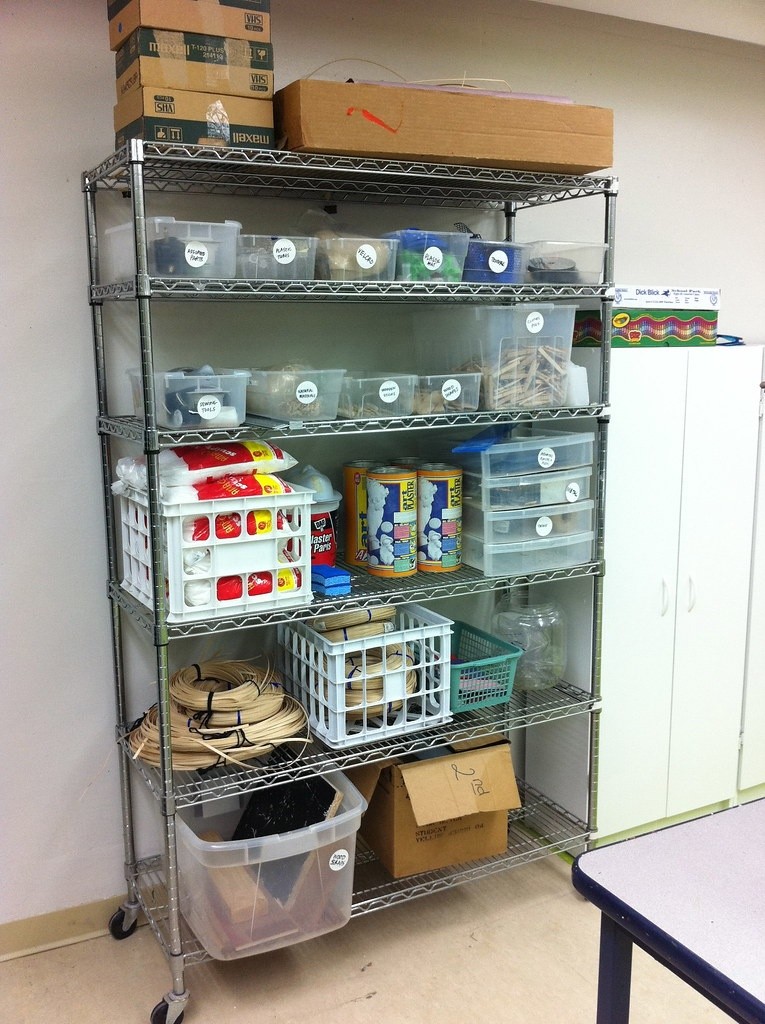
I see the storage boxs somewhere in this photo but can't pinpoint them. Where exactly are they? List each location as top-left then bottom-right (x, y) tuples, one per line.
(276, 602), (455, 749)
(412, 303), (578, 411)
(418, 427), (597, 578)
(241, 367), (348, 422)
(337, 371), (418, 419)
(106, 0), (276, 165)
(120, 480), (318, 624)
(234, 235), (319, 285)
(127, 368), (251, 431)
(317, 239), (399, 284)
(104, 215), (242, 279)
(273, 78), (615, 202)
(463, 240), (534, 284)
(414, 374), (481, 414)
(524, 239), (609, 288)
(379, 230), (473, 284)
(341, 730), (523, 879)
(572, 283), (720, 347)
(177, 744), (369, 961)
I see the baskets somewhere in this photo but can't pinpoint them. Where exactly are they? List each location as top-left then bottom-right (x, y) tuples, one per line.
(396, 620), (523, 713)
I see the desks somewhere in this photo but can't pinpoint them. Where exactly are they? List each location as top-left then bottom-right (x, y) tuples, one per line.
(572, 799), (765, 1024)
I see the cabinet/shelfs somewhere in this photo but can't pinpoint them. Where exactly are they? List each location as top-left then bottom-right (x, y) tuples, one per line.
(524, 346), (765, 858)
(83, 137), (619, 1023)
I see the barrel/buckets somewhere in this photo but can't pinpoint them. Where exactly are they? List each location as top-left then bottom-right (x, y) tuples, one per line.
(491, 590), (567, 690)
